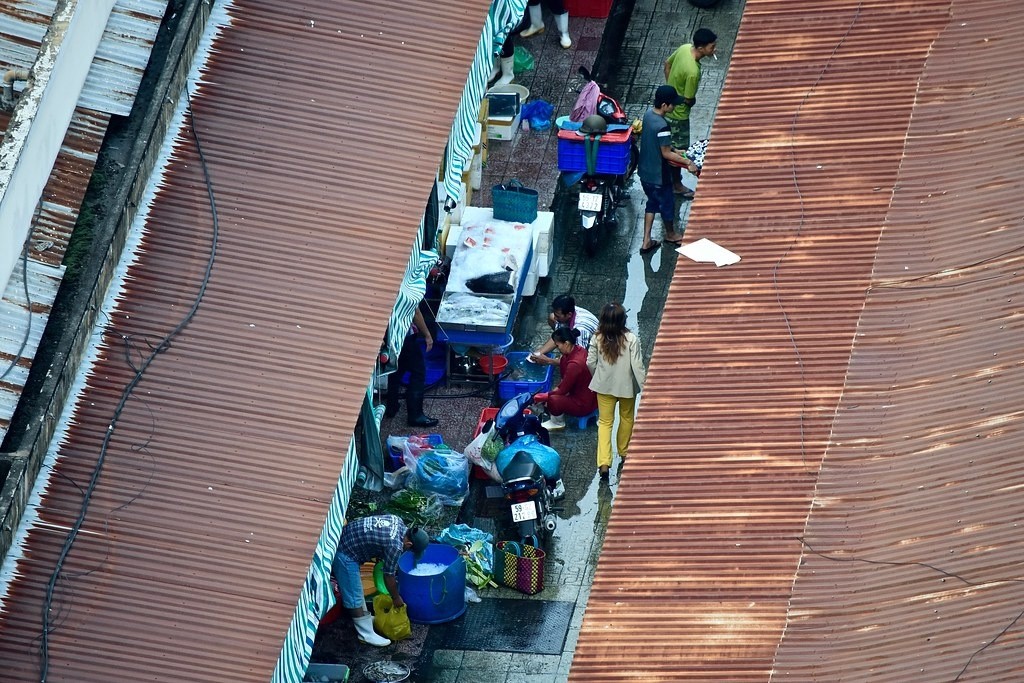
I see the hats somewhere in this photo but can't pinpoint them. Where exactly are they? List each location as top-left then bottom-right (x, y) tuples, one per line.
(411, 528), (429, 559)
(655, 85), (684, 105)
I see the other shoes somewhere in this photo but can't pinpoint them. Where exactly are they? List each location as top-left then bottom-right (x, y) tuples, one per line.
(622, 456), (626, 462)
(600, 464), (609, 477)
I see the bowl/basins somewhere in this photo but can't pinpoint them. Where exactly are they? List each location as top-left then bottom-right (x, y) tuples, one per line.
(479, 355), (508, 374)
(487, 83), (530, 105)
(476, 334), (514, 355)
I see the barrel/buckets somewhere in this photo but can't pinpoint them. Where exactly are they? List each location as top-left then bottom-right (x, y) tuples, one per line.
(396, 543), (467, 624)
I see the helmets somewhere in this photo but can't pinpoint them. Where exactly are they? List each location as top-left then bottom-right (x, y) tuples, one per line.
(579, 115), (607, 133)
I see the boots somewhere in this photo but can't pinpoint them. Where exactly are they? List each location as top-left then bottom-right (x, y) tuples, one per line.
(353, 611), (391, 646)
(488, 55), (501, 84)
(520, 4), (544, 37)
(553, 12), (572, 49)
(406, 390), (438, 427)
(540, 413), (566, 432)
(385, 384), (401, 418)
(361, 597), (375, 624)
(495, 55), (514, 85)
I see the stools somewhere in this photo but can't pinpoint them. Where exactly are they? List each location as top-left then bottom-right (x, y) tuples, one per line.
(576, 408), (600, 430)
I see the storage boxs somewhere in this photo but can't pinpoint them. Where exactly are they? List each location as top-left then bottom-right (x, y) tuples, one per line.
(498, 351), (556, 402)
(564, 0), (613, 19)
(557, 123), (634, 175)
(473, 407), (531, 480)
(439, 92), (554, 296)
(387, 434), (444, 469)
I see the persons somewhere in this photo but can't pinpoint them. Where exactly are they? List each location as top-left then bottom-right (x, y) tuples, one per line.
(338, 514), (429, 646)
(638, 86), (699, 254)
(531, 294), (600, 366)
(586, 302), (646, 477)
(533, 327), (598, 432)
(385, 305), (439, 426)
(486, 31), (514, 86)
(664, 27), (716, 199)
(520, 0), (571, 48)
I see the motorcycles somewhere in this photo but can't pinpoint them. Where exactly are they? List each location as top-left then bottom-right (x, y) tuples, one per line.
(577, 65), (640, 257)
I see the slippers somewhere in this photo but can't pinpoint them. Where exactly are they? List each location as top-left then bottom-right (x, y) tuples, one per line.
(664, 234), (683, 246)
(673, 188), (694, 199)
(640, 240), (661, 254)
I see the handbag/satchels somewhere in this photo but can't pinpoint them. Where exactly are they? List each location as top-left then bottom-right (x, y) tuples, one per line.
(463, 421), (561, 484)
(491, 178), (538, 223)
(374, 594), (411, 641)
(493, 535), (546, 595)
(403, 440), (469, 507)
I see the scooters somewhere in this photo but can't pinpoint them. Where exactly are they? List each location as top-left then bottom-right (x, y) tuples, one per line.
(482, 385), (565, 549)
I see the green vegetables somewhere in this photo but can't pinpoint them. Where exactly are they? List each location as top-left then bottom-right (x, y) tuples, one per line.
(480, 433), (504, 461)
(388, 490), (444, 526)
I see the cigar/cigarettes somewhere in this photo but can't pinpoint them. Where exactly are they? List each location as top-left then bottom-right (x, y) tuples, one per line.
(713, 54), (717, 60)
